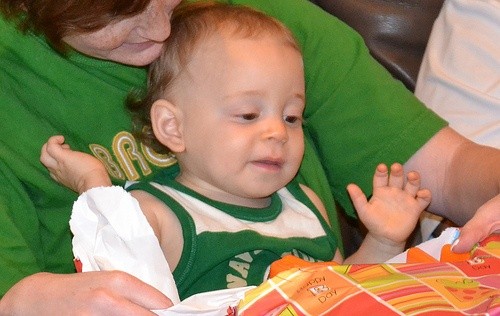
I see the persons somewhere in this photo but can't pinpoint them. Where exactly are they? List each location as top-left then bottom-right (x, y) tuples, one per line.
(38, 3), (432, 308)
(0, 0), (500, 316)
